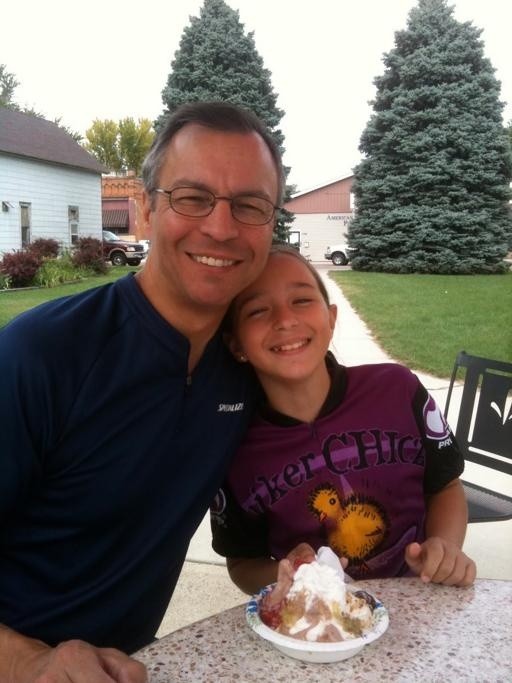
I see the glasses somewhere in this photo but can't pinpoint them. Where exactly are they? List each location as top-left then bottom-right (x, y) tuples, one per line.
(148, 184), (282, 227)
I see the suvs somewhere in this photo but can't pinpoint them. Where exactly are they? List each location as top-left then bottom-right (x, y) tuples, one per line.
(102, 230), (145, 266)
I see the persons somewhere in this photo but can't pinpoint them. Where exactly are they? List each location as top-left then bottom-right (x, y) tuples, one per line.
(1, 100), (286, 681)
(208, 241), (478, 598)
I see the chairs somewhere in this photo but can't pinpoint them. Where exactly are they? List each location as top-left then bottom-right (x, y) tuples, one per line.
(441, 351), (512, 525)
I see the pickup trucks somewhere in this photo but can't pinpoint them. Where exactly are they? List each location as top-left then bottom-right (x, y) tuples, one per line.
(325, 240), (357, 265)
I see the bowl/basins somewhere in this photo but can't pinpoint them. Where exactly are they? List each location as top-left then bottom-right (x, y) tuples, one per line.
(244, 581), (390, 665)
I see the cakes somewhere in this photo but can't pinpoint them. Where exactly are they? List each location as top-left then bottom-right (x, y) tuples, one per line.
(256, 543), (373, 643)
